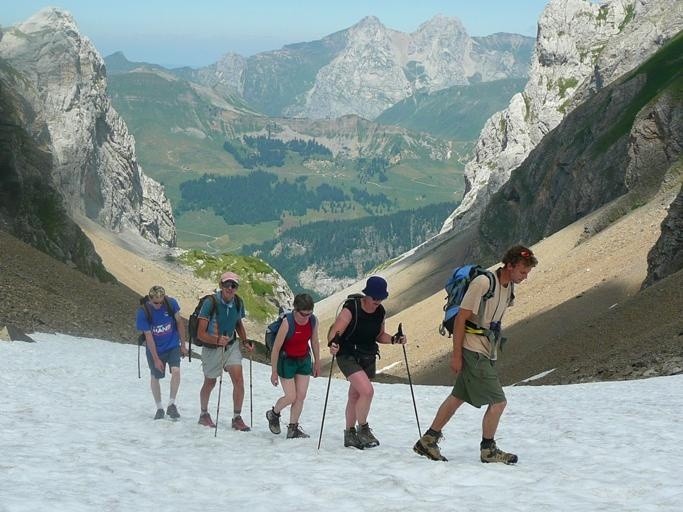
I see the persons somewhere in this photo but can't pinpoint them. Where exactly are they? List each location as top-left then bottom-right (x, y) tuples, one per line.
(413, 245), (538, 465)
(266, 293), (320, 439)
(136, 285), (188, 420)
(197, 272), (254, 431)
(327, 276), (407, 450)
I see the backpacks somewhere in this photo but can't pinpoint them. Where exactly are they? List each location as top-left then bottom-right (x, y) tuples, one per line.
(265, 311), (316, 352)
(440, 264), (515, 335)
(188, 294), (240, 346)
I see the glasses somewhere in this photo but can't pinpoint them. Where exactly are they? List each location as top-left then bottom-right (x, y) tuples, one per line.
(298, 311), (311, 317)
(515, 252), (530, 258)
(224, 283), (235, 289)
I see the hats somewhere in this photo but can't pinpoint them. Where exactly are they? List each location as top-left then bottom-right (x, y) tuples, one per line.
(221, 272), (239, 285)
(362, 277), (388, 299)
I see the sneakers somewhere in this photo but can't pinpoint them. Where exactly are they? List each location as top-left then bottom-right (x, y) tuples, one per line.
(287, 422), (311, 438)
(154, 409), (164, 419)
(413, 434), (448, 462)
(167, 404), (179, 418)
(480, 442), (517, 464)
(232, 416), (250, 431)
(199, 413), (214, 427)
(266, 409), (281, 434)
(344, 423), (379, 450)
